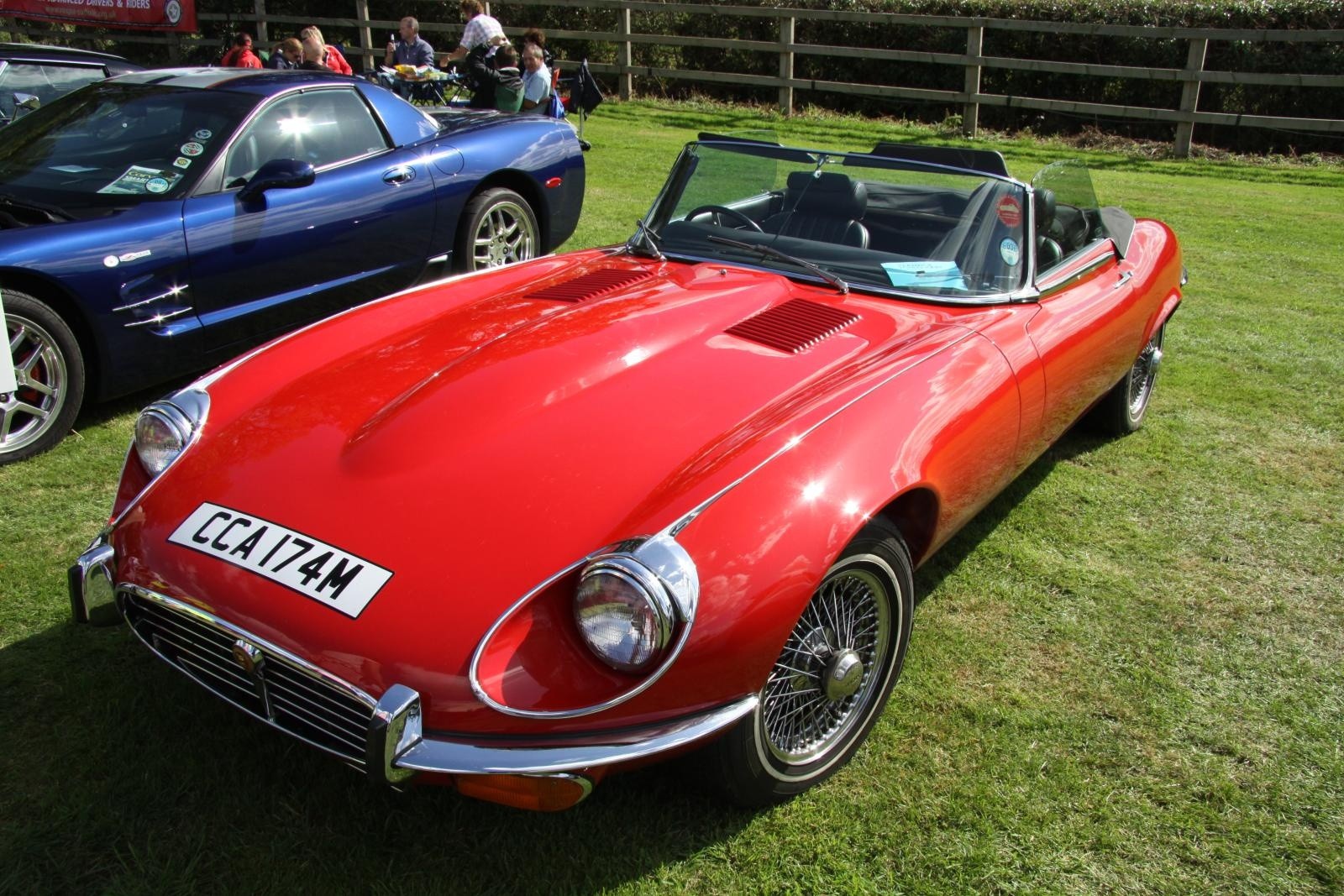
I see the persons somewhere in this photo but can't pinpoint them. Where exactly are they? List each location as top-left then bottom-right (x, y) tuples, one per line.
(440, 0), (554, 114)
(385, 16), (434, 98)
(222, 25), (352, 76)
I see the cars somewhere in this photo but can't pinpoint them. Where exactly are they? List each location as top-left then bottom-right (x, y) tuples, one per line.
(0, 45), (155, 160)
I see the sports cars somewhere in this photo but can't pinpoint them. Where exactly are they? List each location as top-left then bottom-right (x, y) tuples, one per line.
(61, 129), (1190, 816)
(1, 66), (590, 471)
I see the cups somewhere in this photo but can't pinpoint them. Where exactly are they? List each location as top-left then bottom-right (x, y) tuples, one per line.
(432, 73), (458, 81)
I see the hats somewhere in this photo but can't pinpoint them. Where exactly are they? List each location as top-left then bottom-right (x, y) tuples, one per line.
(236, 32), (251, 45)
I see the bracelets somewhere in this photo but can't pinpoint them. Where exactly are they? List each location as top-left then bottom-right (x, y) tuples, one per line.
(447, 55), (451, 62)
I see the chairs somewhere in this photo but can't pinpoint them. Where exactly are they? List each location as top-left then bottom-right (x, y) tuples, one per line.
(930, 181), (1064, 292)
(765, 181), (871, 250)
(178, 105), (256, 177)
(259, 42), (604, 151)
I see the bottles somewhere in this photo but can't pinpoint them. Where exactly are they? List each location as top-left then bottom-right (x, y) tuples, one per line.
(390, 34), (396, 52)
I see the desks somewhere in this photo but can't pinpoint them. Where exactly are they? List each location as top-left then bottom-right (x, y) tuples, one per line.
(378, 65), (466, 106)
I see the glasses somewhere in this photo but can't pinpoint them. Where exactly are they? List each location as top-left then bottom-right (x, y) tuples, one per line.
(291, 52), (301, 59)
(301, 34), (313, 42)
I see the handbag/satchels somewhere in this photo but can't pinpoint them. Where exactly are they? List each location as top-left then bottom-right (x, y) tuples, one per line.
(550, 90), (565, 119)
